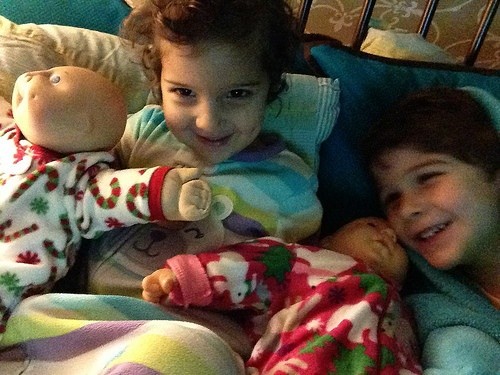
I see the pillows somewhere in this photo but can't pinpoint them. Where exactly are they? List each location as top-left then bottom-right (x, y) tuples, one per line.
(0, 17), (340, 194)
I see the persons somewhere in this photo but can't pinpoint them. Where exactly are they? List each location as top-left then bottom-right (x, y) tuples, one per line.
(0, 66), (212, 334)
(1, 0), (325, 375)
(141, 216), (424, 375)
(358, 83), (500, 375)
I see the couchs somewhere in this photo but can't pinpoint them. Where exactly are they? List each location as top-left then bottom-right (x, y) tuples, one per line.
(0, 1), (500, 375)
